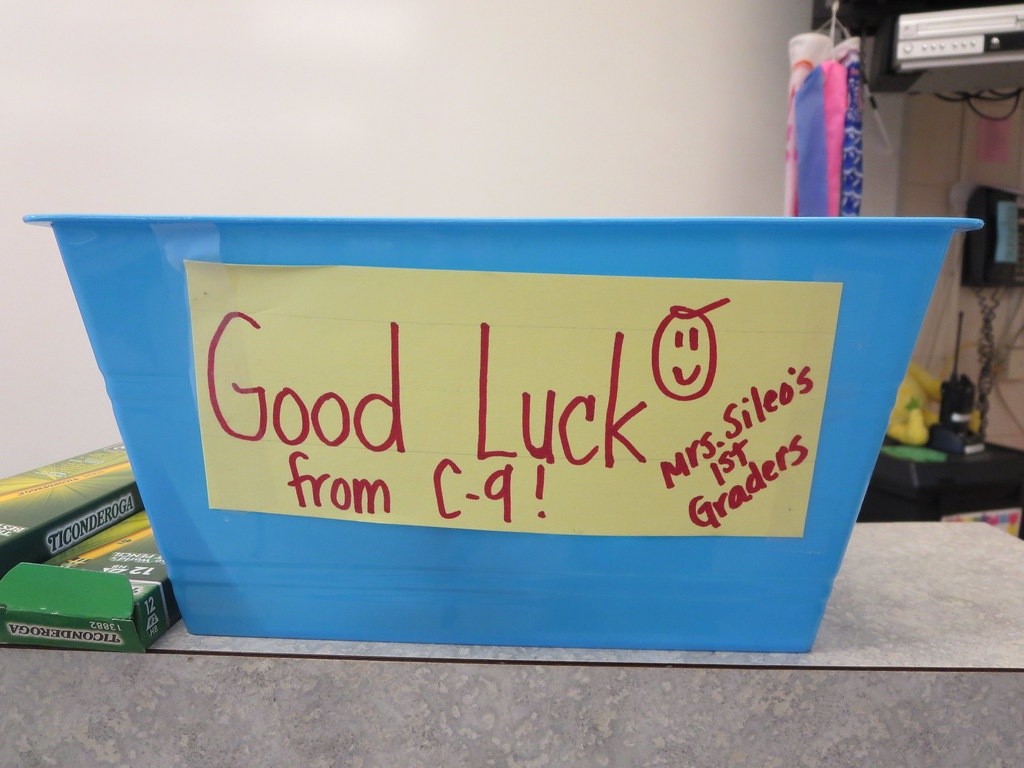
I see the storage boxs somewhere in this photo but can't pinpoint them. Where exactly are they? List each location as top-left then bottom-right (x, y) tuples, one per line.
(0, 510), (182, 653)
(21, 210), (983, 653)
(0, 441), (146, 579)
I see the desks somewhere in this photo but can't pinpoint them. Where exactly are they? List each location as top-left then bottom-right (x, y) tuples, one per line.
(0, 519), (1023, 768)
